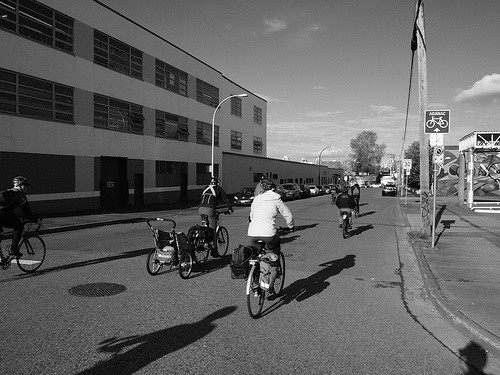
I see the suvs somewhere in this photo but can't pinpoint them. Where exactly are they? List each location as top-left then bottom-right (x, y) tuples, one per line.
(283, 183), (302, 199)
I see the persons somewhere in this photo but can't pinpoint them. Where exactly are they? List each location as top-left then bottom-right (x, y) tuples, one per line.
(197, 176), (234, 258)
(0, 176), (41, 265)
(246, 176), (295, 298)
(336, 181), (360, 229)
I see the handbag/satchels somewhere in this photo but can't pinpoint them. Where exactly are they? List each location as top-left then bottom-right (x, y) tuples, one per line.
(258, 250), (280, 293)
(230, 245), (259, 280)
(187, 225), (214, 244)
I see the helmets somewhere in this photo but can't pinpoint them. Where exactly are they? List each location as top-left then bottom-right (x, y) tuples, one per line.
(13, 176), (31, 187)
(209, 176), (221, 186)
(260, 179), (276, 191)
(261, 176), (267, 180)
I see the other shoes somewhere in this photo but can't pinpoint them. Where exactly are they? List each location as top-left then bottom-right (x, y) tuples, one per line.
(210, 250), (220, 257)
(11, 245), (23, 256)
(347, 224), (352, 229)
(339, 223), (343, 228)
(267, 291), (276, 300)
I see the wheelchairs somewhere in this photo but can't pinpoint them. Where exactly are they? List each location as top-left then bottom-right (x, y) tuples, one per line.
(145, 218), (205, 279)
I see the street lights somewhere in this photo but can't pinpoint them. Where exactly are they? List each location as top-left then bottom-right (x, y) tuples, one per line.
(319, 144), (335, 185)
(210, 93), (249, 183)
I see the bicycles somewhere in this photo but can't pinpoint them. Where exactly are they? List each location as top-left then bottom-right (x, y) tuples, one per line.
(0, 217), (46, 272)
(189, 210), (229, 258)
(331, 194), (337, 206)
(354, 197), (357, 218)
(245, 228), (286, 319)
(339, 206), (352, 239)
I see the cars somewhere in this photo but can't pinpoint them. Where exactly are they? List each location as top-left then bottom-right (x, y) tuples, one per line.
(331, 186), (338, 192)
(300, 185), (311, 198)
(274, 184), (287, 201)
(309, 185), (320, 196)
(317, 186), (326, 195)
(335, 184), (341, 190)
(370, 183), (382, 188)
(381, 181), (397, 196)
(360, 184), (368, 188)
(234, 187), (254, 205)
(325, 186), (331, 194)
